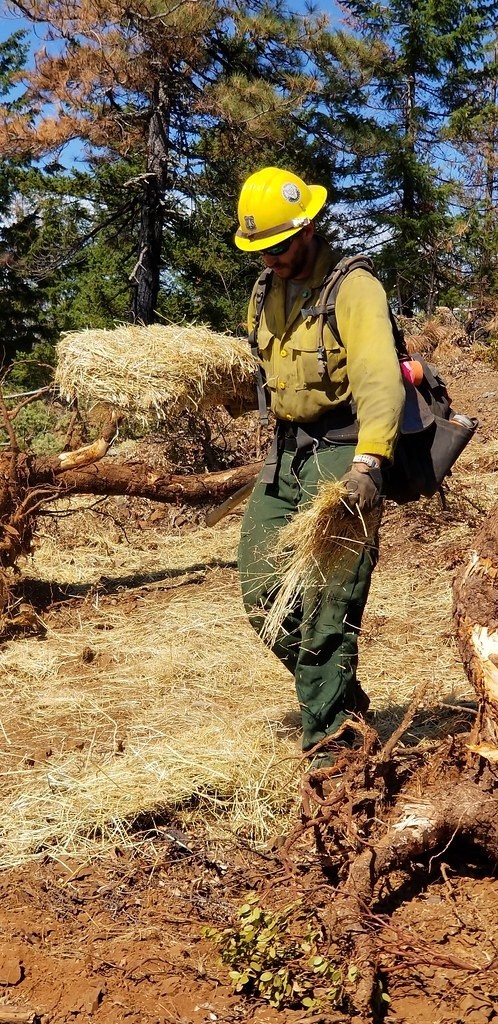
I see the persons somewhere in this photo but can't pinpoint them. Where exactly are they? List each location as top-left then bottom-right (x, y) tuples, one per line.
(231, 168), (406, 770)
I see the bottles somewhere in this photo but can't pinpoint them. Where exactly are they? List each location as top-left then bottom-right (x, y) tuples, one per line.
(451, 414), (479, 430)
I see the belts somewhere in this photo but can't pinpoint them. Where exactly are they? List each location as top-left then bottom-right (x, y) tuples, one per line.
(278, 437), (330, 451)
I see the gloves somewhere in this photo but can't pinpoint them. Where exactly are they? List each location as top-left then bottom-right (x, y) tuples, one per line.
(339, 461), (383, 518)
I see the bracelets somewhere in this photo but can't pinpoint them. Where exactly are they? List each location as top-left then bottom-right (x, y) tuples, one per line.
(351, 455), (380, 472)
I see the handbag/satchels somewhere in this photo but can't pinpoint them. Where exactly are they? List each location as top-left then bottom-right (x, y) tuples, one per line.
(325, 256), (479, 500)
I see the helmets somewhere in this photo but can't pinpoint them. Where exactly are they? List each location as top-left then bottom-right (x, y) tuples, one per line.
(234, 166), (327, 252)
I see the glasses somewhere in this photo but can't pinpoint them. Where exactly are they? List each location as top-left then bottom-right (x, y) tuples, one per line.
(259, 231), (301, 256)
